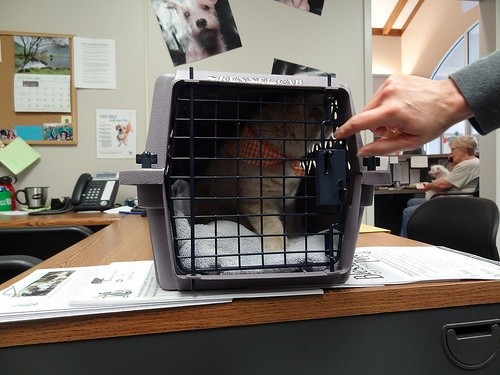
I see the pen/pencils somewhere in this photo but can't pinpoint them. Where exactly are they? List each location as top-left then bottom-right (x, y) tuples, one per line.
(118, 211), (143, 215)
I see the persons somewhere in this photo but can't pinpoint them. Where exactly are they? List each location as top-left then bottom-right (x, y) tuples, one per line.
(399, 135), (480, 238)
(8, 130), (16, 139)
(333, 48), (500, 157)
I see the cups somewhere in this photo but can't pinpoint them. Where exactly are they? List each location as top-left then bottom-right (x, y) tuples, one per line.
(15, 187), (48, 209)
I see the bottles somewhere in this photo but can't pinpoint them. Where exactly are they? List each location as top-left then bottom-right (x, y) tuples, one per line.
(0, 187), (12, 212)
(0, 175), (17, 210)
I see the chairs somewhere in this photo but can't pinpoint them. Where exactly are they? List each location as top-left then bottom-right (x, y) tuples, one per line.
(406, 194), (499, 262)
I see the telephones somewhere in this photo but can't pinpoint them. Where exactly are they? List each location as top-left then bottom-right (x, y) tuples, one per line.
(69, 171), (120, 212)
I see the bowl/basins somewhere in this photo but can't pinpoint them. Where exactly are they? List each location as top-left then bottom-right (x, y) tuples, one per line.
(416, 182), (428, 189)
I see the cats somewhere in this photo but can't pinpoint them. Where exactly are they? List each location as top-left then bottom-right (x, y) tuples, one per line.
(200, 99), (336, 253)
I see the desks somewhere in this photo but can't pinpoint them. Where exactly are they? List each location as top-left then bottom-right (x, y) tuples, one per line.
(0, 209), (500, 375)
(0, 205), (128, 228)
(374, 182), (479, 195)
